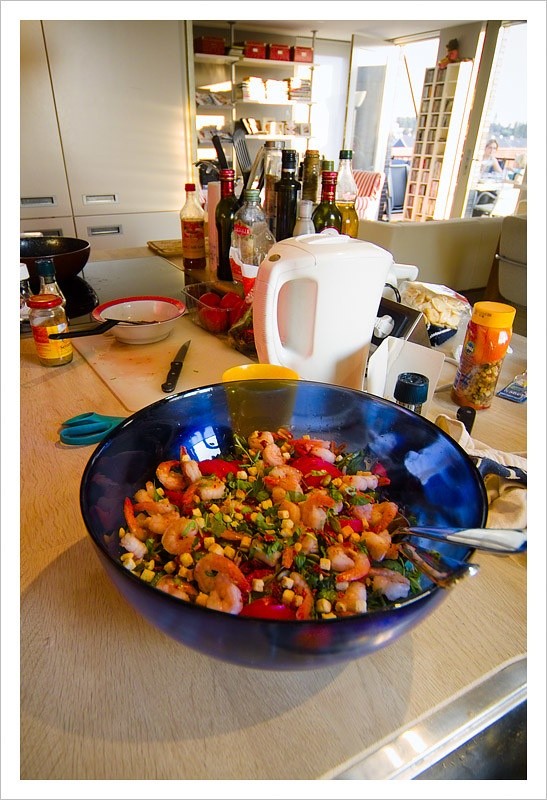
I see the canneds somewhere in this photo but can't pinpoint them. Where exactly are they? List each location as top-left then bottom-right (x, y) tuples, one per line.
(450, 301), (516, 411)
(27, 294), (73, 367)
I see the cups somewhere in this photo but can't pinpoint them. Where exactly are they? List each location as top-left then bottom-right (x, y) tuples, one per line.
(223, 364), (299, 439)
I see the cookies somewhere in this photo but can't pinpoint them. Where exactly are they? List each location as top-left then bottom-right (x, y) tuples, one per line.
(404, 287), (461, 330)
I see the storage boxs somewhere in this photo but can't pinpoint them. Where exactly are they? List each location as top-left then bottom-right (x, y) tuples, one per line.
(236, 38), (313, 63)
(194, 35), (226, 56)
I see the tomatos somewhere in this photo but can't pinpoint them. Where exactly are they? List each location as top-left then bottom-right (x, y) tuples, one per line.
(196, 291), (249, 333)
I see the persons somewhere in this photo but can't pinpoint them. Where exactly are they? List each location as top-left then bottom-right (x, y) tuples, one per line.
(472, 139), (526, 216)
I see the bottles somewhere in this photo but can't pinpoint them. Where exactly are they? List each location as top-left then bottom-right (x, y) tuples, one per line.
(311, 171), (342, 235)
(316, 160), (334, 204)
(274, 149), (301, 242)
(35, 259), (70, 325)
(451, 301), (516, 410)
(229, 189), (276, 299)
(394, 372), (429, 415)
(215, 169), (241, 282)
(180, 183), (206, 270)
(301, 150), (319, 216)
(292, 200), (316, 236)
(335, 149), (359, 239)
(262, 141), (286, 242)
(20, 263), (35, 332)
(26, 294), (73, 367)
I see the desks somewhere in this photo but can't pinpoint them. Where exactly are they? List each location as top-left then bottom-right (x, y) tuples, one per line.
(475, 180), (516, 202)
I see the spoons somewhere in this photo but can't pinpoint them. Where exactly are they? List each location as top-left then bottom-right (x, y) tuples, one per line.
(387, 513), (481, 591)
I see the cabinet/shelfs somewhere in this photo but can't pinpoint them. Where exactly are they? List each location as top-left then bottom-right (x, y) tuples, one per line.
(194, 22), (317, 181)
(20, 20), (200, 250)
(400, 62), (474, 219)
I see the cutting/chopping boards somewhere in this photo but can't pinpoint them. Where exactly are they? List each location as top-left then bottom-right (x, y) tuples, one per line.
(147, 237), (209, 258)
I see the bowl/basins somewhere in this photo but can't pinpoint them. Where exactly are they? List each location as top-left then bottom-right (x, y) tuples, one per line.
(181, 282), (249, 334)
(92, 295), (186, 345)
(80, 378), (488, 670)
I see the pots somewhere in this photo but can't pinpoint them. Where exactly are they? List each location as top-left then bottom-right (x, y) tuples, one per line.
(20, 236), (91, 277)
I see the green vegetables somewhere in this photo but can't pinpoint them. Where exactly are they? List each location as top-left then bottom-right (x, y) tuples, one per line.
(135, 432), (442, 619)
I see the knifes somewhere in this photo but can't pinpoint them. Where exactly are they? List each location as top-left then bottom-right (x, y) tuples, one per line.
(161, 340), (191, 393)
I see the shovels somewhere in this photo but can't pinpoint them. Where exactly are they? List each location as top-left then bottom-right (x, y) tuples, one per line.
(232, 129), (252, 200)
(48, 319), (159, 340)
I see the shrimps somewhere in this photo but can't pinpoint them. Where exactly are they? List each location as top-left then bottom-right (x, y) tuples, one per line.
(124, 427), (410, 618)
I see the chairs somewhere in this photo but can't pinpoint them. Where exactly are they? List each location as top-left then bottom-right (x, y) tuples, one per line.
(473, 185), (522, 217)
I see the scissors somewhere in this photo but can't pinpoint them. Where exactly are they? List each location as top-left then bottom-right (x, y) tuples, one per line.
(60, 412), (127, 445)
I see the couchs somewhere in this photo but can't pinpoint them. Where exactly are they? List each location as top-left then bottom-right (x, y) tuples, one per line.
(358, 217), (506, 302)
(351, 170), (387, 219)
(495, 213), (527, 310)
